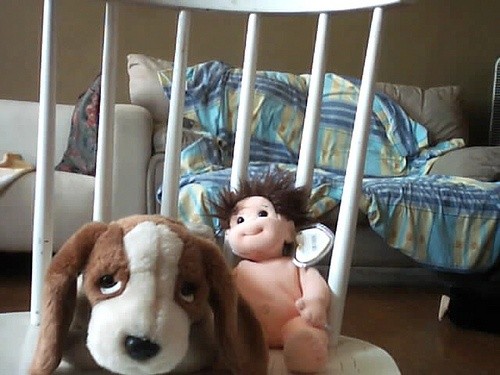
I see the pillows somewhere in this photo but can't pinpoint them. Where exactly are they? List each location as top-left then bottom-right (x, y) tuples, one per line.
(422, 146), (500, 182)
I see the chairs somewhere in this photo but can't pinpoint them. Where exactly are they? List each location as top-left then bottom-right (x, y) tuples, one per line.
(0, 0), (406, 375)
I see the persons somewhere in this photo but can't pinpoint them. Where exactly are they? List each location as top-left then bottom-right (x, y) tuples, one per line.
(201, 169), (330, 375)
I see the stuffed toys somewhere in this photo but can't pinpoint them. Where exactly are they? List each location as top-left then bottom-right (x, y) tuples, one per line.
(25, 214), (269, 375)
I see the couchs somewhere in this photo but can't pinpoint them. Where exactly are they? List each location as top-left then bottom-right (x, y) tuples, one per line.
(0, 53), (500, 297)
